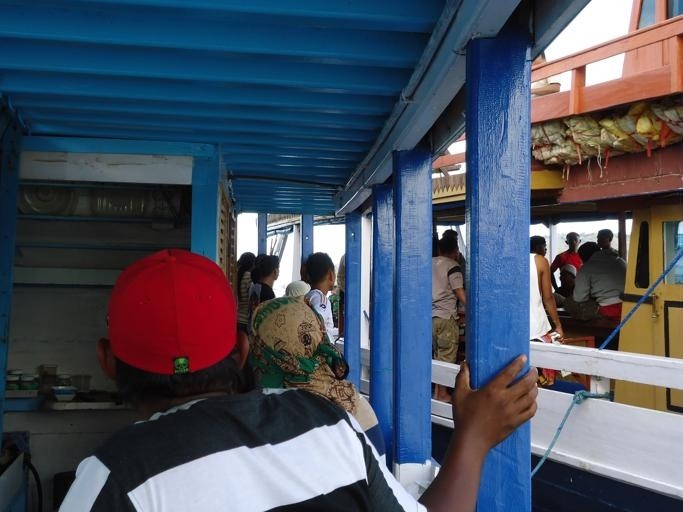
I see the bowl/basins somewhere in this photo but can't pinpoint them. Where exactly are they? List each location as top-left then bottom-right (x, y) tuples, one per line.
(51, 386), (79, 402)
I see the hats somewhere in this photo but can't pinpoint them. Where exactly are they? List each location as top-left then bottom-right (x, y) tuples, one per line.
(104, 246), (240, 375)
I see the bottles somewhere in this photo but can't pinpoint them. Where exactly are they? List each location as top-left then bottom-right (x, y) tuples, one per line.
(5, 368), (39, 392)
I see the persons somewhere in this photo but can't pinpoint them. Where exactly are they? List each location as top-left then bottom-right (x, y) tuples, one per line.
(285, 252), (346, 344)
(432, 228), (469, 403)
(54, 249), (543, 511)
(234, 253), (280, 395)
(529, 229), (627, 346)
(248, 298), (387, 464)
(529, 51), (563, 99)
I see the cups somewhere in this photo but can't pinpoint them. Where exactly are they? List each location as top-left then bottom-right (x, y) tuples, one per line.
(59, 375), (71, 386)
